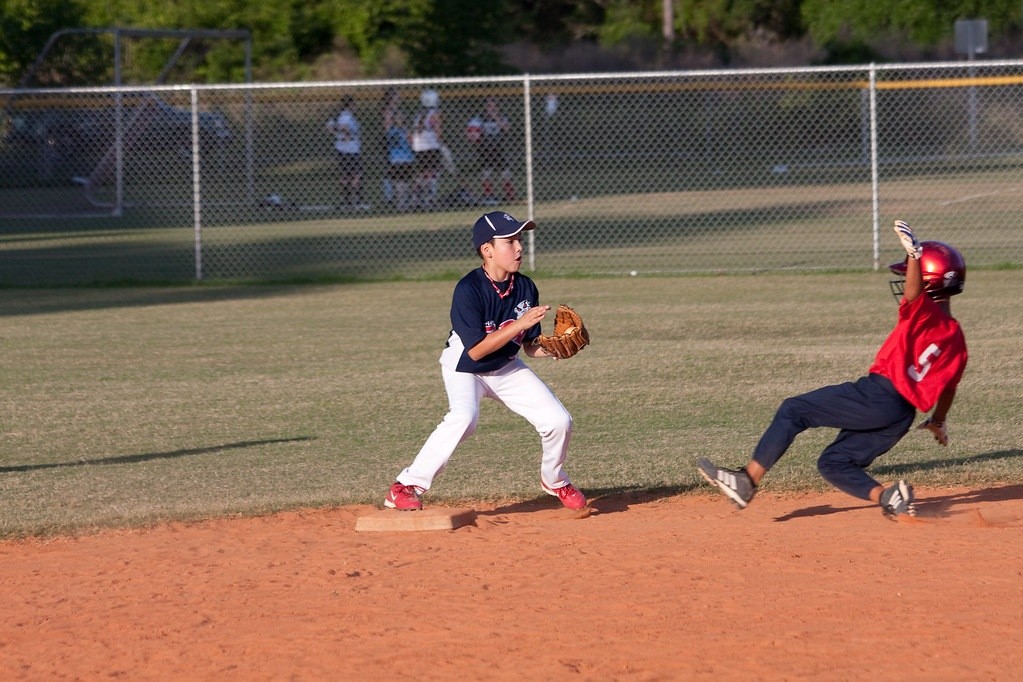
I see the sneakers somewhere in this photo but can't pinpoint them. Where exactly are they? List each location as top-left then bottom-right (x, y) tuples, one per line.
(383, 482), (423, 510)
(695, 454), (759, 510)
(879, 480), (918, 524)
(540, 476), (587, 511)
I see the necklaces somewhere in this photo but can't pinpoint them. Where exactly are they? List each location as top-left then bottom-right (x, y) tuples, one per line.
(483, 265), (515, 299)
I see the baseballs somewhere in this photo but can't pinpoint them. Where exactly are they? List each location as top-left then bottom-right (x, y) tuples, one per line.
(564, 327), (577, 334)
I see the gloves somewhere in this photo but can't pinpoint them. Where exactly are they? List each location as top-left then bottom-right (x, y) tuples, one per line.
(914, 417), (949, 448)
(893, 219), (923, 260)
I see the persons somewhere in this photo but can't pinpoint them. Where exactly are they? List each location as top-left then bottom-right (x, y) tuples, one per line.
(696, 219), (967, 517)
(466, 97), (516, 201)
(380, 87), (452, 212)
(326, 94), (363, 213)
(384, 211), (590, 511)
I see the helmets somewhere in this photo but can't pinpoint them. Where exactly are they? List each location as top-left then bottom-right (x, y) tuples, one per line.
(420, 89), (441, 109)
(888, 240), (966, 295)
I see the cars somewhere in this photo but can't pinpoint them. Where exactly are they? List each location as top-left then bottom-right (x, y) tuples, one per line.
(0, 81), (248, 193)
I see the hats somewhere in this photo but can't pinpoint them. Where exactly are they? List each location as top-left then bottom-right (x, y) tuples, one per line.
(472, 210), (536, 252)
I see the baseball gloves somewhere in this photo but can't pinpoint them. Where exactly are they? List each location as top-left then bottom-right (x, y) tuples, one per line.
(538, 305), (590, 360)
